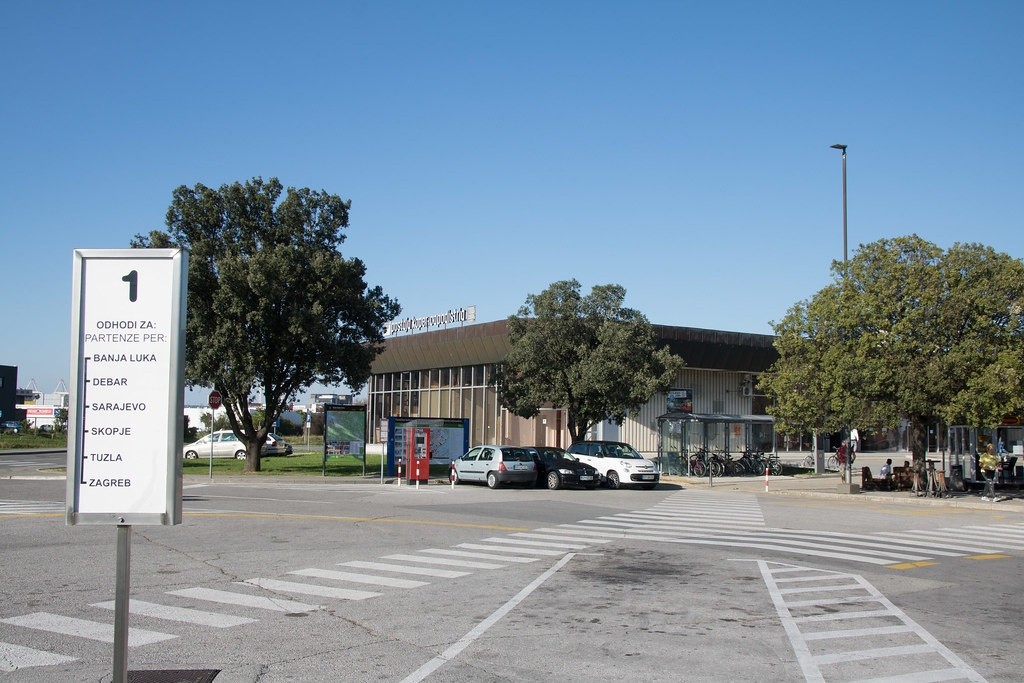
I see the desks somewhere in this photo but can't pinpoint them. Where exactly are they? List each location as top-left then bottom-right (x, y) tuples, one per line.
(893, 466), (913, 491)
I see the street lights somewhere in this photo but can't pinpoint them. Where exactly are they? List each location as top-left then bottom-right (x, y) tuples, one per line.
(830, 144), (847, 264)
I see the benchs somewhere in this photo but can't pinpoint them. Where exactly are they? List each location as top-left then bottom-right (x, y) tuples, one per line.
(862, 467), (886, 491)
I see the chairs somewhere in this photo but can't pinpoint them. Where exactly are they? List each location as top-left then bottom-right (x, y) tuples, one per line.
(515, 452), (523, 457)
(998, 456), (1018, 483)
(617, 448), (623, 457)
(547, 453), (554, 458)
(504, 452), (509, 457)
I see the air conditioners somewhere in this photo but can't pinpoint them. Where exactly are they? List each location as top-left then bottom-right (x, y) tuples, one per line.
(738, 386), (753, 398)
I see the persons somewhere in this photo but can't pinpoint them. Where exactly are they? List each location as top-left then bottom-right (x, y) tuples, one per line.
(880, 459), (892, 480)
(904, 461), (909, 467)
(979, 443), (1001, 503)
(839, 440), (857, 484)
(851, 428), (859, 452)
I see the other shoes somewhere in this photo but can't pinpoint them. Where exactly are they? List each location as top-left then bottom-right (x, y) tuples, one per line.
(981, 496), (990, 501)
(993, 497), (1002, 502)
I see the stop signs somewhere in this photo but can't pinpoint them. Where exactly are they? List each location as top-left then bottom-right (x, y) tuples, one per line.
(209, 390), (222, 410)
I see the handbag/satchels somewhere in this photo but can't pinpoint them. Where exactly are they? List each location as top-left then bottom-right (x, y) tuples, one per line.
(985, 469), (995, 478)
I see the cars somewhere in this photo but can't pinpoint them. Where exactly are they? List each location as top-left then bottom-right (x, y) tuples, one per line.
(448, 445), (538, 490)
(508, 446), (602, 490)
(564, 441), (660, 490)
(0, 420), (23, 434)
(182, 429), (268, 460)
(224, 430), (294, 457)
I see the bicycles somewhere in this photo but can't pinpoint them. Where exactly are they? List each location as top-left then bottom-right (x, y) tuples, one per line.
(827, 443), (856, 470)
(803, 445), (815, 467)
(670, 445), (783, 478)
